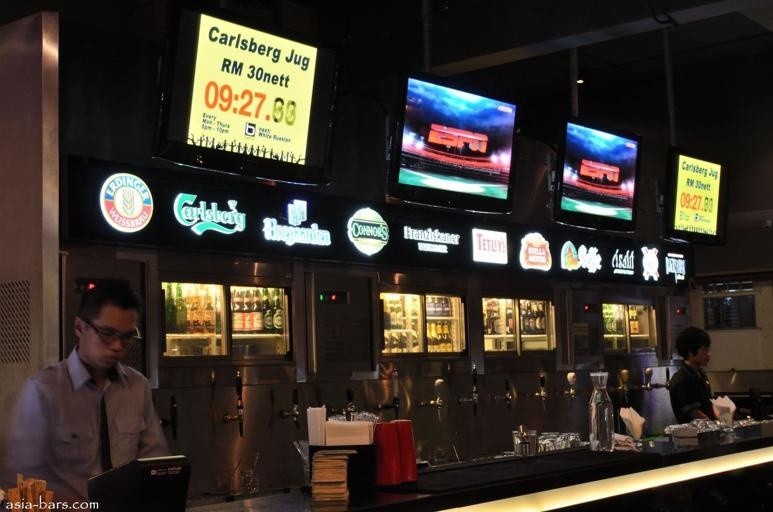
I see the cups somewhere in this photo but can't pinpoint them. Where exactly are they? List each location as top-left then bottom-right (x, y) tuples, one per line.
(719, 414), (733, 433)
(667, 418), (758, 434)
(537, 432), (580, 450)
(306, 407), (325, 445)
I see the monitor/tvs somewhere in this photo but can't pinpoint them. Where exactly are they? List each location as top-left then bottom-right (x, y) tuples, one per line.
(153, 0), (347, 188)
(385, 71), (522, 215)
(551, 115), (642, 233)
(658, 147), (731, 247)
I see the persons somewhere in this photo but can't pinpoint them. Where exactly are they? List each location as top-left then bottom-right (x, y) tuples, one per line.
(0, 281), (173, 509)
(667, 325), (718, 425)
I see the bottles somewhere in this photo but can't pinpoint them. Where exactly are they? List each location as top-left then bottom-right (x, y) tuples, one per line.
(163, 282), (286, 355)
(482, 298), (545, 335)
(587, 370), (615, 450)
(380, 294), (453, 354)
(603, 304), (640, 334)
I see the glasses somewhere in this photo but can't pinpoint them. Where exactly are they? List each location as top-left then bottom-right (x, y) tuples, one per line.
(75, 313), (144, 348)
